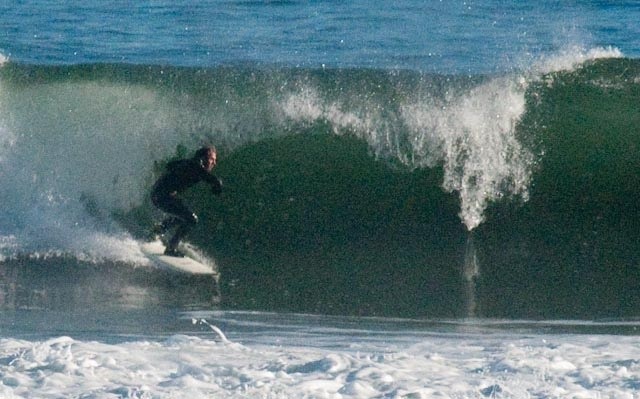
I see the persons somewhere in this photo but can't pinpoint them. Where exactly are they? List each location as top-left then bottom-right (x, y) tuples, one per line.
(151, 145), (224, 259)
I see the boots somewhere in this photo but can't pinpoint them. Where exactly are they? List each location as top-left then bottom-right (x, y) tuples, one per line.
(164, 244), (185, 258)
(146, 230), (163, 243)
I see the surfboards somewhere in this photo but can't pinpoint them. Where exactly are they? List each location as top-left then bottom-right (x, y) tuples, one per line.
(140, 245), (217, 274)
